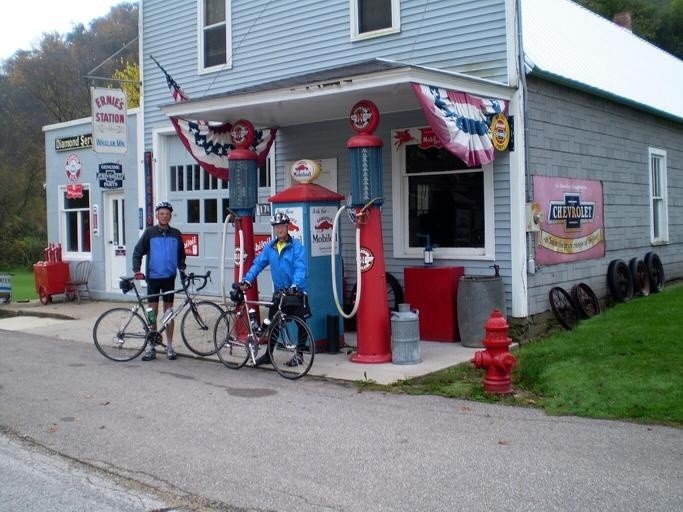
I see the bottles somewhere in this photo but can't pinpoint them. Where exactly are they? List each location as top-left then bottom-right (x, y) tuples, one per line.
(160, 306), (174, 323)
(247, 309), (258, 330)
(146, 308), (155, 323)
(255, 319), (270, 338)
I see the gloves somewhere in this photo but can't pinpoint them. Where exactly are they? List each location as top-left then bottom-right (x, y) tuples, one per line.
(134, 271), (145, 280)
(179, 270), (187, 280)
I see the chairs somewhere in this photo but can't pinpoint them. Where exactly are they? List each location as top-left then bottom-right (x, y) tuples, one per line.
(63, 260), (93, 306)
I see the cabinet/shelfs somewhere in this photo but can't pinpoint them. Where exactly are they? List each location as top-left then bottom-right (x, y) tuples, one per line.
(403, 267), (464, 343)
(32, 262), (75, 305)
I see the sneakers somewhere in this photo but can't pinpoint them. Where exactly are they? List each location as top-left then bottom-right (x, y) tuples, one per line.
(140, 346), (177, 361)
(251, 352), (304, 366)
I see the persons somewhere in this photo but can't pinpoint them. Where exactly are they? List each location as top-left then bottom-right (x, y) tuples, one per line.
(130, 201), (187, 362)
(238, 212), (309, 368)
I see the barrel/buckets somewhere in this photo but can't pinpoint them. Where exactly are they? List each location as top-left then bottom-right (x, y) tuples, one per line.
(390, 304), (421, 365)
(458, 275), (507, 347)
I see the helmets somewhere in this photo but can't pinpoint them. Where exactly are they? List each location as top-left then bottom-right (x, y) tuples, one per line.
(154, 201), (173, 213)
(268, 212), (290, 226)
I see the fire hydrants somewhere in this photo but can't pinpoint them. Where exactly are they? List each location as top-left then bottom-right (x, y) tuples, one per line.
(470, 308), (516, 396)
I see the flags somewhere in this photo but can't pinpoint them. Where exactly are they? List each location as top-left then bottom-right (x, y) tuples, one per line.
(163, 74), (207, 126)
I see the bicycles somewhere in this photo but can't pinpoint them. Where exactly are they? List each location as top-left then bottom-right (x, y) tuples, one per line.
(93, 271), (314, 380)
